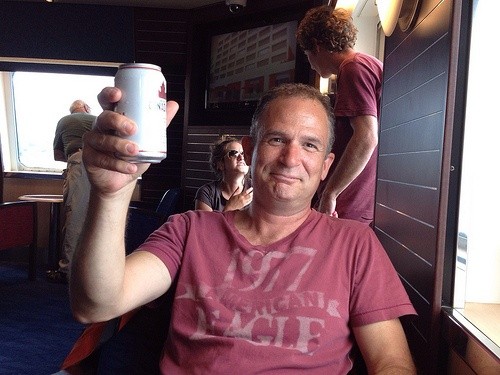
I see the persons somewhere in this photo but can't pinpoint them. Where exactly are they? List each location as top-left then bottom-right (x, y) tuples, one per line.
(297, 5), (383, 224)
(194, 135), (253, 211)
(44, 99), (98, 285)
(66, 83), (418, 375)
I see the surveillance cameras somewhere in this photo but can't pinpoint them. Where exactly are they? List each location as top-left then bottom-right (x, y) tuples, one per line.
(226, 0), (246, 14)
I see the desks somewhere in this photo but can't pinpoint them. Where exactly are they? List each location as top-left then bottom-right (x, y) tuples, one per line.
(18, 195), (66, 282)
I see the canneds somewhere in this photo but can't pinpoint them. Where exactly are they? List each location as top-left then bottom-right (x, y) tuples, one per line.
(113, 63), (167, 163)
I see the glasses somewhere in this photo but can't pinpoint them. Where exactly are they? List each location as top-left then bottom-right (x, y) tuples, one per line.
(225, 150), (245, 159)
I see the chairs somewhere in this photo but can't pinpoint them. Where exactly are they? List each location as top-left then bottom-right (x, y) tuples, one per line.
(0, 200), (41, 281)
(129, 187), (180, 236)
